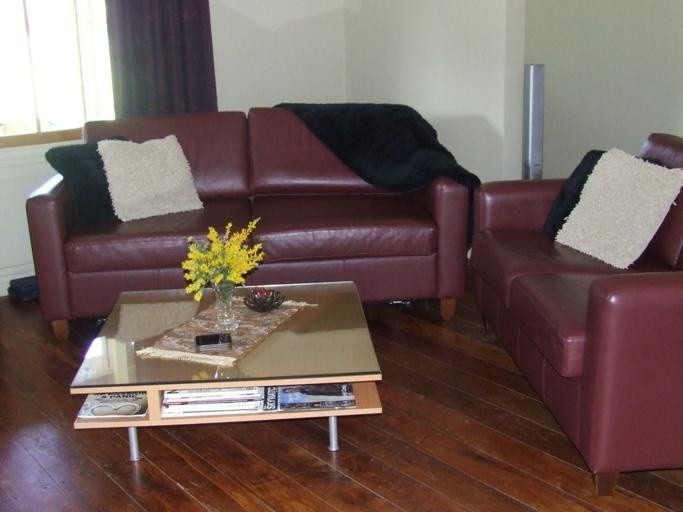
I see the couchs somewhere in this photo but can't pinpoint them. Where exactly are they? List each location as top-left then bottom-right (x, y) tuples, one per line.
(466, 128), (682, 496)
(24, 104), (468, 341)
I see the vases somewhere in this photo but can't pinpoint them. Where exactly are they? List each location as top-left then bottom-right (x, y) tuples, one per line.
(212, 280), (238, 333)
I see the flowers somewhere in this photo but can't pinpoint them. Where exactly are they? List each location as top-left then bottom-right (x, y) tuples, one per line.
(179, 212), (266, 305)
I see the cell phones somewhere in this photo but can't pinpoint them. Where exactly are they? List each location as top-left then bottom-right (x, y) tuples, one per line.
(196, 333), (233, 352)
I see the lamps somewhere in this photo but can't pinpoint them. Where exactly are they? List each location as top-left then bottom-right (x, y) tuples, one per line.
(521, 64), (545, 181)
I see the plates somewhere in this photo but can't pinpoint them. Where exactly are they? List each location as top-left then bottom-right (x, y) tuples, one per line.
(244, 288), (287, 311)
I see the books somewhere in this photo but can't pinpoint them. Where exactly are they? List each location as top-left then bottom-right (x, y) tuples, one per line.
(75, 379), (358, 421)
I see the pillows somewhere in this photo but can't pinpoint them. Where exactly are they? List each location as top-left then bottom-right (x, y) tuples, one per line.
(94, 133), (206, 222)
(542, 150), (659, 237)
(549, 147), (682, 270)
(44, 134), (126, 229)
